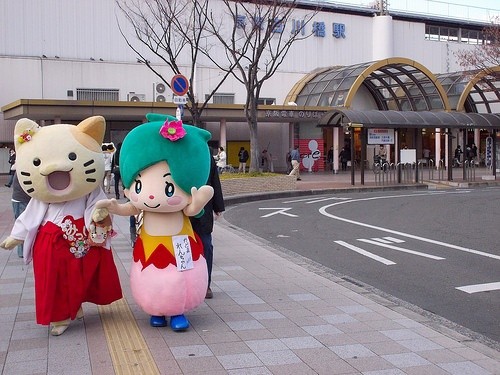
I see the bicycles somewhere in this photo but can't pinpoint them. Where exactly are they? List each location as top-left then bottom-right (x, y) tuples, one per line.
(213, 155), (236, 175)
(373, 156), (390, 174)
(394, 154), (487, 170)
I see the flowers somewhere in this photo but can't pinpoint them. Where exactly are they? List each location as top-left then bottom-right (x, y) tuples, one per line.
(159, 118), (188, 141)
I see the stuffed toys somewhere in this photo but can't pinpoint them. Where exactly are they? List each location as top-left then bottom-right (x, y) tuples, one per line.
(0, 116), (125, 337)
(98, 113), (215, 334)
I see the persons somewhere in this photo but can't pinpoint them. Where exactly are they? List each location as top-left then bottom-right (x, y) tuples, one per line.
(454, 143), (477, 168)
(215, 147), (227, 174)
(328, 146), (333, 172)
(238, 147), (249, 173)
(288, 145), (300, 181)
(189, 156), (227, 300)
(4, 149), (16, 187)
(339, 147), (347, 173)
(100, 152), (112, 195)
(111, 141), (128, 199)
(12, 168), (31, 258)
(376, 145), (386, 171)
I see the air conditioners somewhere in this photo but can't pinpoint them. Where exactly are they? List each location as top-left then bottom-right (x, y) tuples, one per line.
(153, 82), (174, 103)
(127, 93), (146, 103)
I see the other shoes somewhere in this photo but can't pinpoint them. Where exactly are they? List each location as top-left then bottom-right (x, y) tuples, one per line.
(170, 314), (189, 330)
(150, 315), (167, 327)
(76, 307), (84, 320)
(204, 287), (213, 299)
(50, 323), (68, 336)
